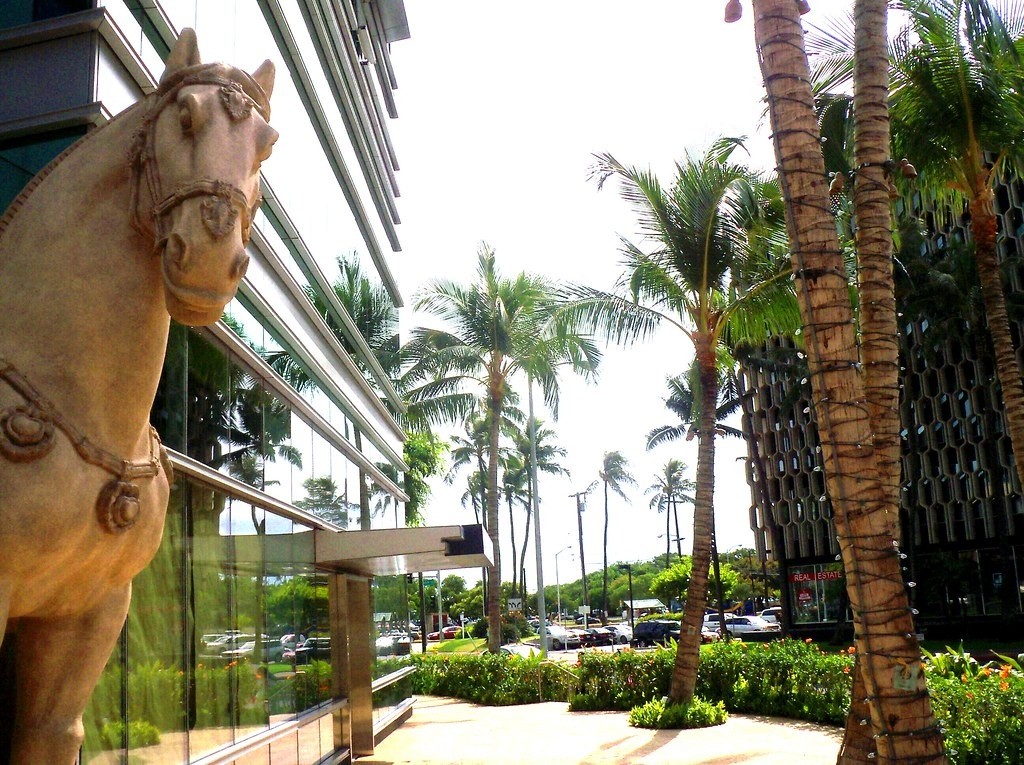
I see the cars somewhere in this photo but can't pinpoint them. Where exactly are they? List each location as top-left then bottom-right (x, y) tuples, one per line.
(281, 642), (304, 664)
(427, 625), (463, 640)
(576, 616), (601, 625)
(700, 625), (721, 644)
(411, 631), (419, 642)
(760, 608), (781, 624)
(200, 632), (270, 655)
(715, 616), (781, 640)
(531, 620), (633, 651)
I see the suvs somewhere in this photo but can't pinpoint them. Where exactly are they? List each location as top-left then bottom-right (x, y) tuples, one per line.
(630, 621), (681, 647)
(221, 640), (284, 664)
(294, 637), (332, 663)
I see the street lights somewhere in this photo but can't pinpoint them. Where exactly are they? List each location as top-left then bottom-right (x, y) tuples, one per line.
(555, 545), (572, 626)
(617, 564), (635, 636)
(657, 533), (684, 562)
(726, 543), (743, 564)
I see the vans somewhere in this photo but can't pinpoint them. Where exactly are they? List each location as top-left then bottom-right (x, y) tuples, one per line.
(703, 612), (738, 632)
(279, 634), (306, 647)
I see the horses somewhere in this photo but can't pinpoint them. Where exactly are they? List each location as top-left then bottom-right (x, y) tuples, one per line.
(0, 30), (279, 765)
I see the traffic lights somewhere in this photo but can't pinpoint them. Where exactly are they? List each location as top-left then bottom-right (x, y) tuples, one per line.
(430, 595), (436, 608)
(407, 573), (414, 584)
